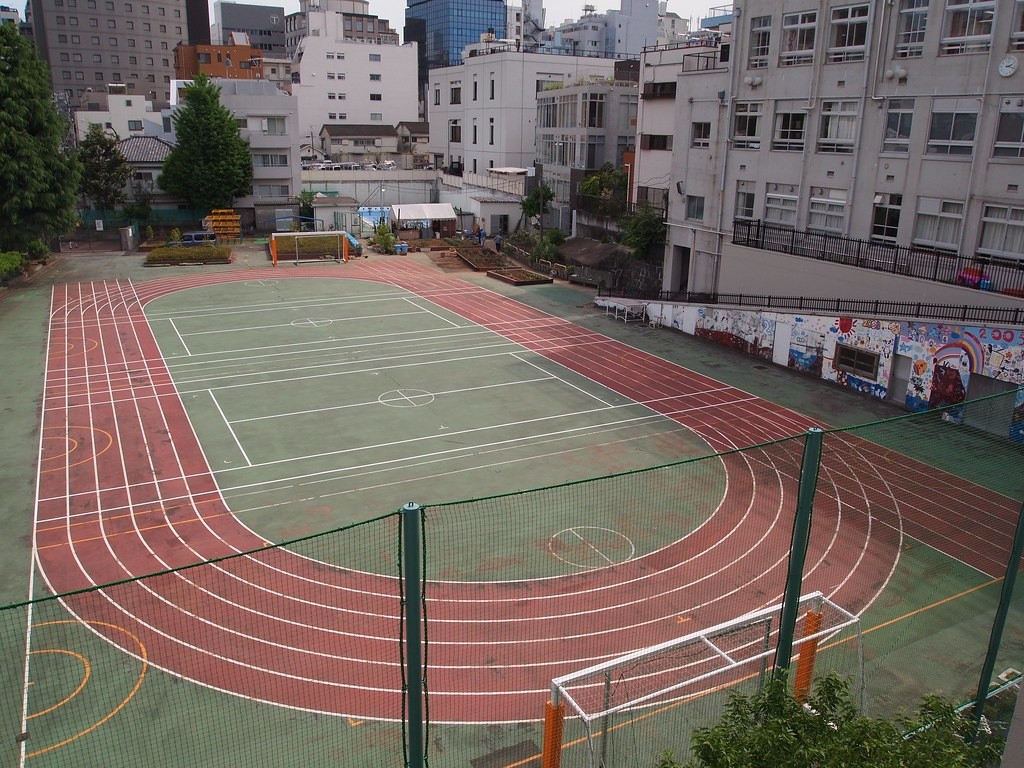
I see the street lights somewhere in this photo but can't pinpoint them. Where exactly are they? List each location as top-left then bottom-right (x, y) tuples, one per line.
(624, 163), (630, 212)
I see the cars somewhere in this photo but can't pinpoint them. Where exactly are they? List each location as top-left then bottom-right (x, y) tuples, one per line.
(302, 159), (396, 170)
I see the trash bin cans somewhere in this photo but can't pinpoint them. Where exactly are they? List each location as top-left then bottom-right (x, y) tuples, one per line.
(394, 243), (401, 254)
(401, 244), (408, 254)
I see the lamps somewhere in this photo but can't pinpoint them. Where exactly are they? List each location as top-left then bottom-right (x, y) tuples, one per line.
(789, 185), (793, 191)
(819, 189), (822, 194)
(773, 184), (777, 190)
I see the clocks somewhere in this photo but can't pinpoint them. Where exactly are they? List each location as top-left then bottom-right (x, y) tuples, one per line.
(998, 55), (1019, 77)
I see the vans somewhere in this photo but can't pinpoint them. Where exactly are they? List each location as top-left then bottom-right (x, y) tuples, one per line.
(167, 231), (217, 248)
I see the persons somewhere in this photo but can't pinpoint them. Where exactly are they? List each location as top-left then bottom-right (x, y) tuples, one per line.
(473, 227), (504, 253)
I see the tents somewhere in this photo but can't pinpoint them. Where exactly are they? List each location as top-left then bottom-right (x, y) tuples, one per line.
(388, 203), (457, 240)
